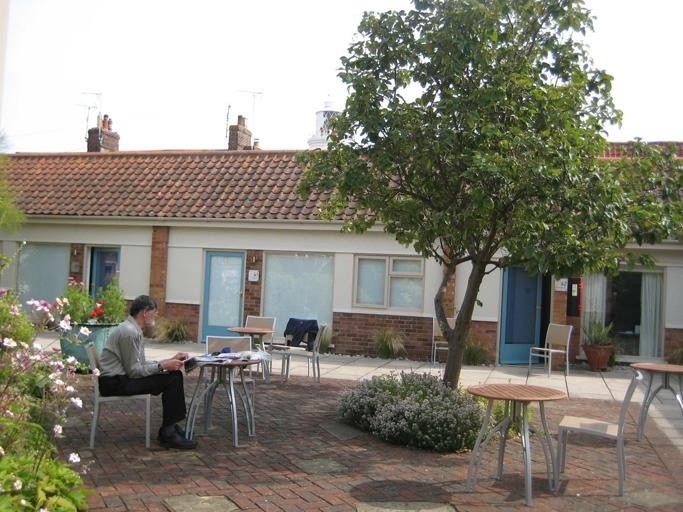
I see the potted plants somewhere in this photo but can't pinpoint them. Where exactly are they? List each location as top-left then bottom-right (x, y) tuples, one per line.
(580, 319), (621, 372)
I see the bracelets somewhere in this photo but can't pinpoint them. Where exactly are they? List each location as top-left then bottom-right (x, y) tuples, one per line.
(158, 363), (163, 371)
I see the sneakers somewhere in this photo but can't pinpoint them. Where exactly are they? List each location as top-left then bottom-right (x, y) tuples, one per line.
(156, 423), (197, 441)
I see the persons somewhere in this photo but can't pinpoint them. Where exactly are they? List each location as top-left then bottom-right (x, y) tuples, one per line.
(98, 295), (197, 449)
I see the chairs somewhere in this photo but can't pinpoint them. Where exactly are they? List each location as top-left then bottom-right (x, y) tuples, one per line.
(552, 368), (641, 497)
(227, 312), (326, 383)
(429, 316), (455, 364)
(80, 339), (150, 449)
(203, 334), (257, 436)
(527, 320), (573, 378)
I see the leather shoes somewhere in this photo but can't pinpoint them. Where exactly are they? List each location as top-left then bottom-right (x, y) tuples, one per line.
(160, 431), (198, 450)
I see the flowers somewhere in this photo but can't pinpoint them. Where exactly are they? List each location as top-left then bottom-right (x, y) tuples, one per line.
(53, 272), (128, 322)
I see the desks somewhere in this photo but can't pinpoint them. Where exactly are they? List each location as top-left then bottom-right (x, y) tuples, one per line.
(459, 384), (566, 507)
(627, 361), (682, 443)
(184, 355), (264, 443)
